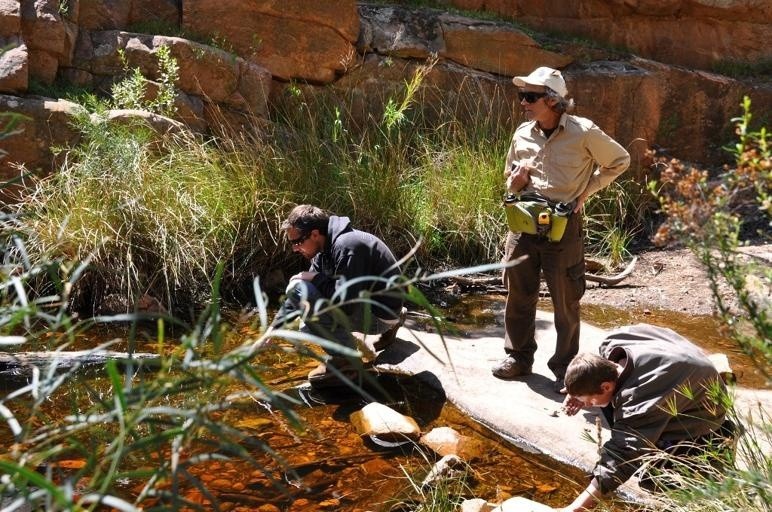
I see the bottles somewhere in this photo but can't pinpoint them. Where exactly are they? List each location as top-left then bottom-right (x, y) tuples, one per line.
(553, 200), (575, 216)
(505, 192), (517, 207)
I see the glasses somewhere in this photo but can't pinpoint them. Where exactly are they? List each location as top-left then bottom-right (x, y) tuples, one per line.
(291, 234), (310, 245)
(516, 91), (551, 103)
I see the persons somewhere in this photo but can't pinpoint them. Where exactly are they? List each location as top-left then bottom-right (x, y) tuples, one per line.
(490, 64), (632, 396)
(258, 203), (412, 391)
(557, 322), (731, 511)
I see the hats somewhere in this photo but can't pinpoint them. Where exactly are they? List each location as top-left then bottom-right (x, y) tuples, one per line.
(511, 67), (568, 98)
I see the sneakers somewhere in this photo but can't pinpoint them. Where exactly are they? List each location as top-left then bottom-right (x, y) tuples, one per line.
(553, 377), (564, 393)
(308, 356), (364, 382)
(491, 356), (532, 379)
(373, 306), (407, 351)
